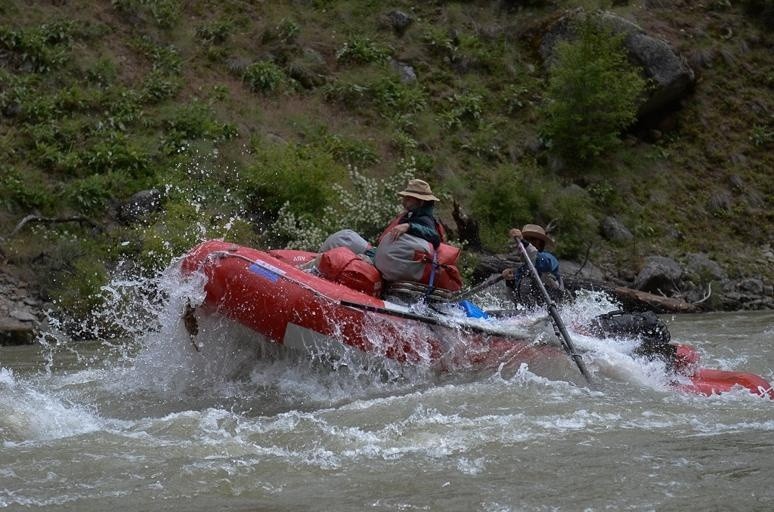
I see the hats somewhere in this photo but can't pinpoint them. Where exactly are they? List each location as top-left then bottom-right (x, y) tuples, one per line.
(398, 178), (441, 202)
(521, 224), (554, 243)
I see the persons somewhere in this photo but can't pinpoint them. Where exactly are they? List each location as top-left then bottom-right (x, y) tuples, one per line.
(377, 178), (440, 248)
(503, 224), (564, 308)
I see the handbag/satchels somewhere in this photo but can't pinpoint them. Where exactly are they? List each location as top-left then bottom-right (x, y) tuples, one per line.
(318, 229), (376, 258)
(318, 247), (383, 299)
(374, 231), (463, 291)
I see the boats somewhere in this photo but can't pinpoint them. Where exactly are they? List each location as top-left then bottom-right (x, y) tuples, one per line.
(180, 239), (774, 403)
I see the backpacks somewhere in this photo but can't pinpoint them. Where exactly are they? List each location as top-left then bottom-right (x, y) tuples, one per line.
(592, 310), (670, 344)
